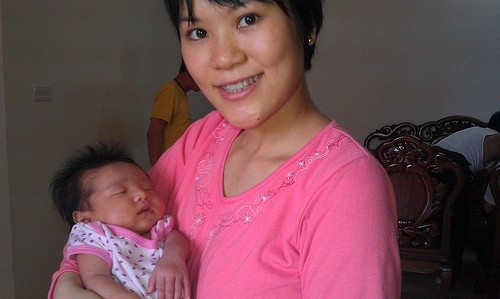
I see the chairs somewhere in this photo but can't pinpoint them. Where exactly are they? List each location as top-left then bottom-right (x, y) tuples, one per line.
(368, 137), (469, 299)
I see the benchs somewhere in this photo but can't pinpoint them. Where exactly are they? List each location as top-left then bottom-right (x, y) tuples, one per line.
(364, 115), (488, 151)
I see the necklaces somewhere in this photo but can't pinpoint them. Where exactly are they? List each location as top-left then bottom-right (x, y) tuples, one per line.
(174, 77), (185, 92)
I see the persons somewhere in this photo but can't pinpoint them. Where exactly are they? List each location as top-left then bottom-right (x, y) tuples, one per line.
(46, 0), (400, 299)
(146, 56), (201, 167)
(429, 111), (500, 283)
(51, 138), (191, 299)
